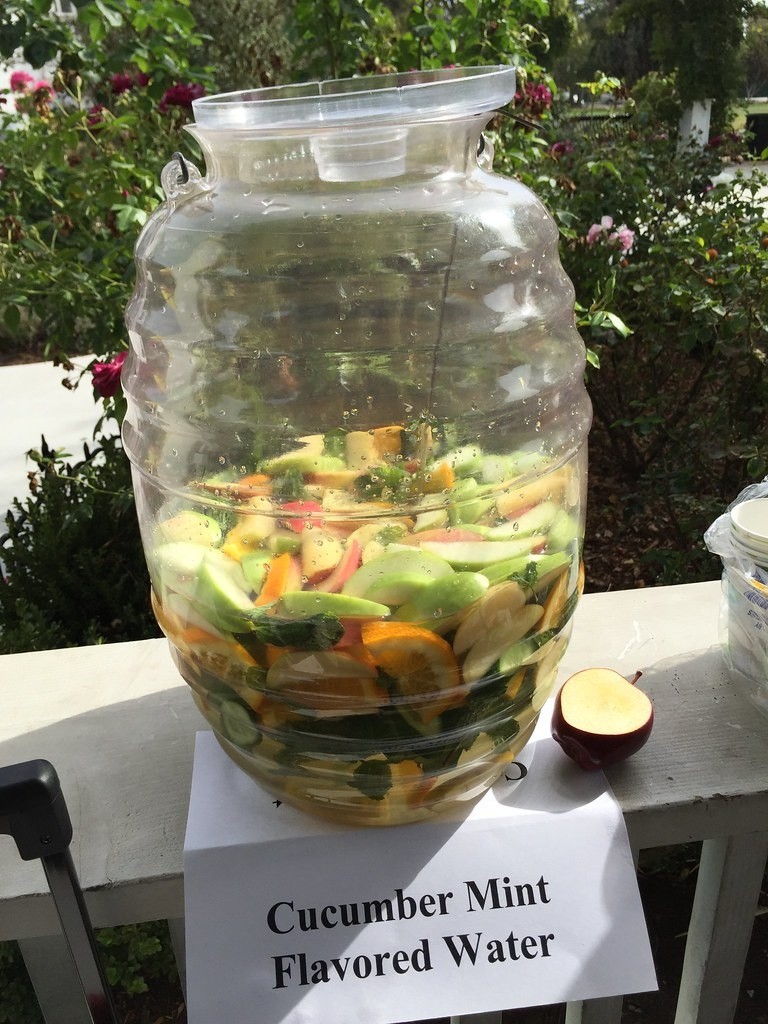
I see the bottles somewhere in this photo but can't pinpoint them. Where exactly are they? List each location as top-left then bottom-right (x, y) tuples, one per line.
(129, 67), (592, 828)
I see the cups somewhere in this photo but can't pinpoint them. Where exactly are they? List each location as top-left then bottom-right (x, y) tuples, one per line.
(730, 497), (768, 682)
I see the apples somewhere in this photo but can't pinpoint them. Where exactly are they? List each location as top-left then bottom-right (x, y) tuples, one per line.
(550, 669), (654, 772)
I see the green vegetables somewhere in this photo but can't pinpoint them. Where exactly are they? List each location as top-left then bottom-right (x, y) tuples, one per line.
(281, 655), (531, 801)
(507, 563), (539, 603)
(243, 605), (344, 650)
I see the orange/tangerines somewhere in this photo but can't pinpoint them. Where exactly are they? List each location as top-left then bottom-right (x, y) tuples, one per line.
(361, 623), (459, 723)
